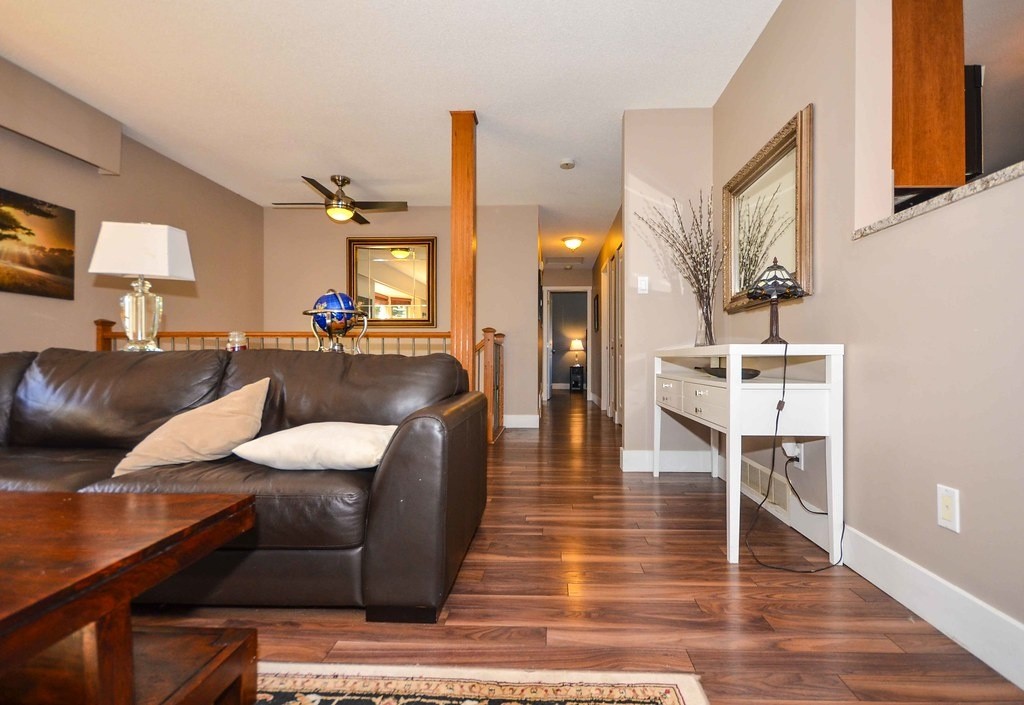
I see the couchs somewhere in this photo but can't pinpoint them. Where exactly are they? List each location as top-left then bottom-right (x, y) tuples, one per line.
(0, 336), (495, 630)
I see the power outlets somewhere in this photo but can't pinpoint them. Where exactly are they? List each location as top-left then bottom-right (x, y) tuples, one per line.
(936, 482), (966, 534)
(793, 443), (805, 471)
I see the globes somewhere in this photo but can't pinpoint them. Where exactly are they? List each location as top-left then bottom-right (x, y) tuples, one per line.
(313, 288), (360, 351)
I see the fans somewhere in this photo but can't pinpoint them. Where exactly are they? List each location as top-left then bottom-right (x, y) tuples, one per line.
(265, 173), (409, 225)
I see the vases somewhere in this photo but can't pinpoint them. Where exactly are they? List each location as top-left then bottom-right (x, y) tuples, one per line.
(693, 292), (717, 346)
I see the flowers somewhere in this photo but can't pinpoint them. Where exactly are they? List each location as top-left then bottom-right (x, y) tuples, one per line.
(630, 182), (730, 295)
(737, 182), (796, 288)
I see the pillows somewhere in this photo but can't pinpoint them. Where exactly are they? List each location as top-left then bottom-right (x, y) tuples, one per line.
(107, 372), (270, 477)
(230, 417), (401, 477)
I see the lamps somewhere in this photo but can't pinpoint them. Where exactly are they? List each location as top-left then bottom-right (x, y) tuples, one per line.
(85, 221), (196, 354)
(568, 338), (586, 367)
(390, 248), (411, 259)
(561, 236), (586, 252)
(745, 255), (809, 344)
(326, 205), (355, 223)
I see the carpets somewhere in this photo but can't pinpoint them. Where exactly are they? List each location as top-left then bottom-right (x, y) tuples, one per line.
(252, 658), (716, 705)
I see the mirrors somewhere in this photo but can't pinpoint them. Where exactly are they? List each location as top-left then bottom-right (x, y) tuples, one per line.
(720, 101), (817, 315)
(345, 236), (437, 328)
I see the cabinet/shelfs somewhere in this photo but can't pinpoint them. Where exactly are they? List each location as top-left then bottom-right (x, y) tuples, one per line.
(893, 0), (986, 196)
(569, 366), (584, 393)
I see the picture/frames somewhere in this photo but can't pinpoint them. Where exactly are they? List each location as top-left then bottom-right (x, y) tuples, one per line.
(593, 294), (600, 332)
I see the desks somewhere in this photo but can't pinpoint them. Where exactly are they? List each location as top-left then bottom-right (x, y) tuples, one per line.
(650, 343), (848, 570)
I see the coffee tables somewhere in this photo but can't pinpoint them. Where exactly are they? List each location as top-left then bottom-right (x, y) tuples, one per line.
(0, 484), (262, 705)
